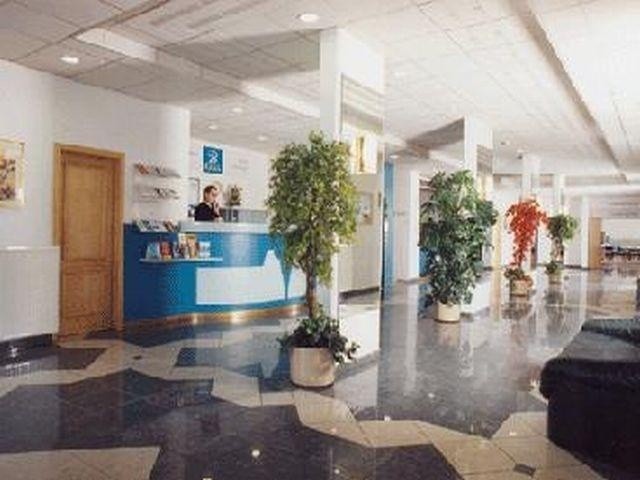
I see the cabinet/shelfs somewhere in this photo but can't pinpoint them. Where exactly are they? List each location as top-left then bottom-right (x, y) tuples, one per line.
(136, 162), (223, 264)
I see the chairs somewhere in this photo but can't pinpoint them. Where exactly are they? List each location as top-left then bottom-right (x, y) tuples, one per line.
(536, 317), (640, 480)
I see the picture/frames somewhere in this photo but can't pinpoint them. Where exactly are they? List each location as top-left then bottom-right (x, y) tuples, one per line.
(0, 138), (26, 209)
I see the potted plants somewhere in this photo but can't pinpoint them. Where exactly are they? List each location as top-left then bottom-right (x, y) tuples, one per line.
(416, 170), (577, 324)
(268, 129), (359, 390)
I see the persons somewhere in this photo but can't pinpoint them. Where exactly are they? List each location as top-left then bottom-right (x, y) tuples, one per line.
(193, 186), (220, 220)
